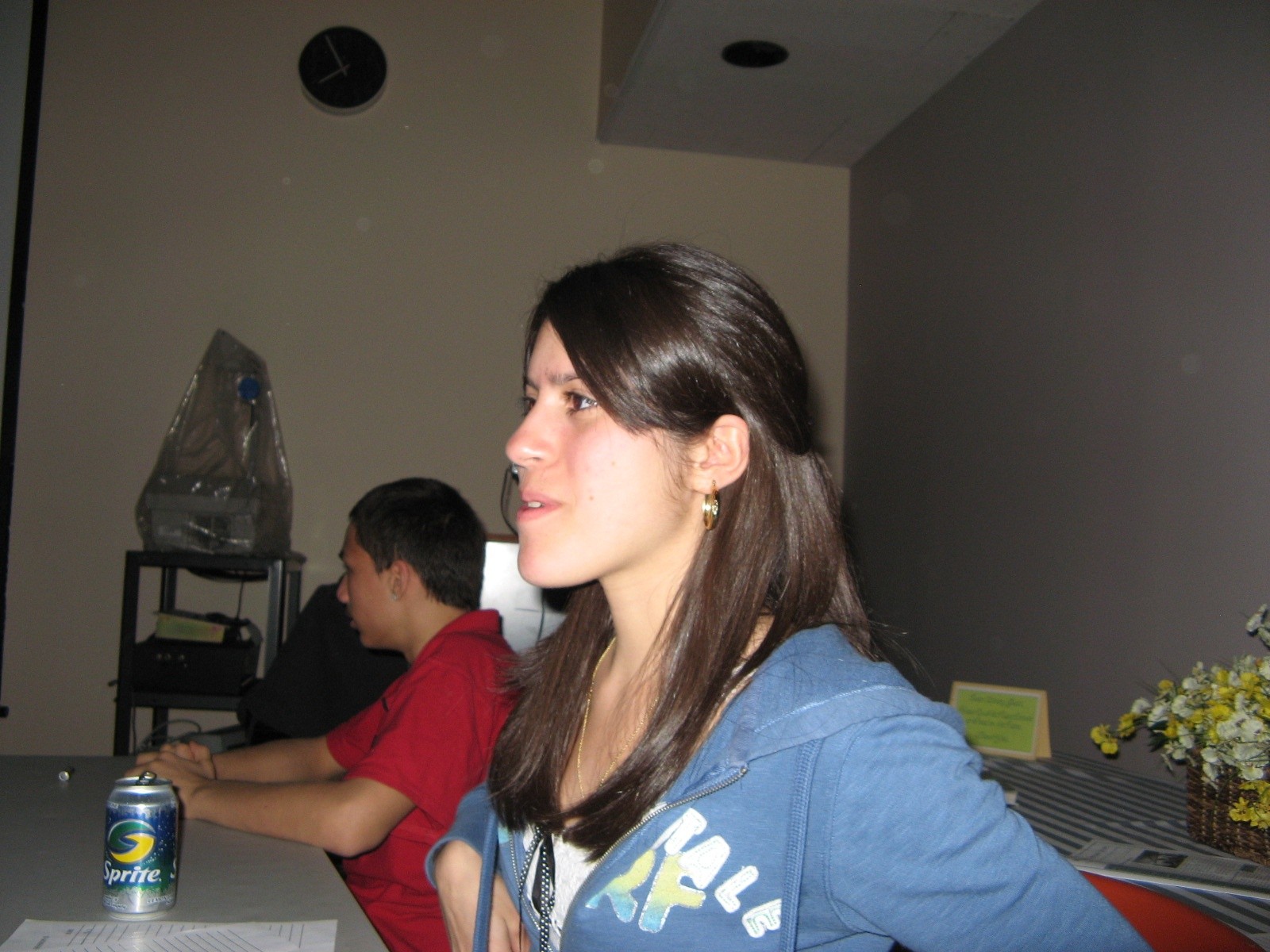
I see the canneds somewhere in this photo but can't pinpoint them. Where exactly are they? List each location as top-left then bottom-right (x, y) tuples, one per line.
(101, 771), (179, 919)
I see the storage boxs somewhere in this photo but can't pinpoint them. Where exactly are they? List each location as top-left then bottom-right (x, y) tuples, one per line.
(145, 494), (283, 554)
(135, 609), (261, 696)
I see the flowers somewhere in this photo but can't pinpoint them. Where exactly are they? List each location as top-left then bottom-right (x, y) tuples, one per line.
(1090, 603), (1270, 829)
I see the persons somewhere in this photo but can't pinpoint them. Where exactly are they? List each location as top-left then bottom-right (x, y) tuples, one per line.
(423, 240), (1154, 952)
(127, 478), (520, 952)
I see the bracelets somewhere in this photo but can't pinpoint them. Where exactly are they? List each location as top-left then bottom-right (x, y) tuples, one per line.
(210, 760), (217, 779)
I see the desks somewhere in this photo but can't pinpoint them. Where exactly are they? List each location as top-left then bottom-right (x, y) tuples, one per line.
(0, 755), (388, 951)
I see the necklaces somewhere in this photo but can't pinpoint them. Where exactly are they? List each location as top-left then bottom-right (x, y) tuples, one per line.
(578, 635), (659, 802)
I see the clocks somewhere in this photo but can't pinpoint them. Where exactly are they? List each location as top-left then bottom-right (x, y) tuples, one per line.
(298, 26), (388, 115)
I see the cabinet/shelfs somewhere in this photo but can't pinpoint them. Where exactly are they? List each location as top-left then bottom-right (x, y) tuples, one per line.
(114, 550), (306, 757)
